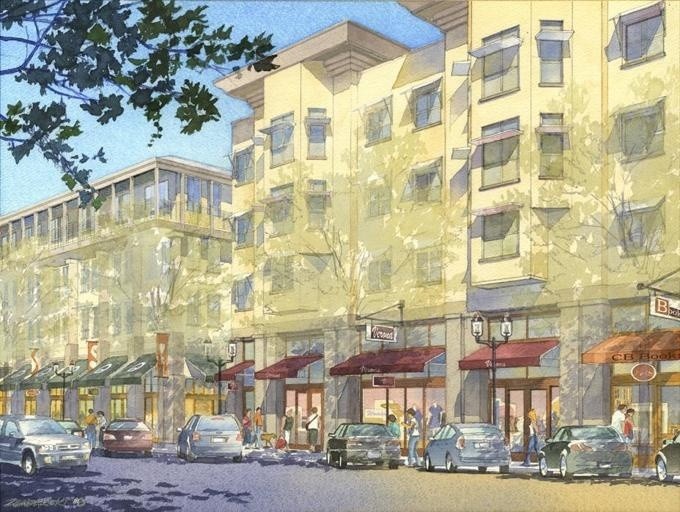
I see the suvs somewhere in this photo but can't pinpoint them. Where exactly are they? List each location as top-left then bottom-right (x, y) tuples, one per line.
(423, 422), (511, 475)
(1, 415), (92, 473)
(175, 413), (243, 465)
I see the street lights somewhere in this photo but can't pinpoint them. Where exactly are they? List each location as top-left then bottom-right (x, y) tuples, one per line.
(52, 361), (75, 420)
(469, 311), (512, 425)
(203, 337), (236, 415)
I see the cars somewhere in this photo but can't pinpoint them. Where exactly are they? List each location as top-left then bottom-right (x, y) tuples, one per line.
(655, 427), (680, 482)
(55, 419), (86, 441)
(101, 416), (154, 457)
(328, 422), (402, 469)
(539, 424), (633, 481)
